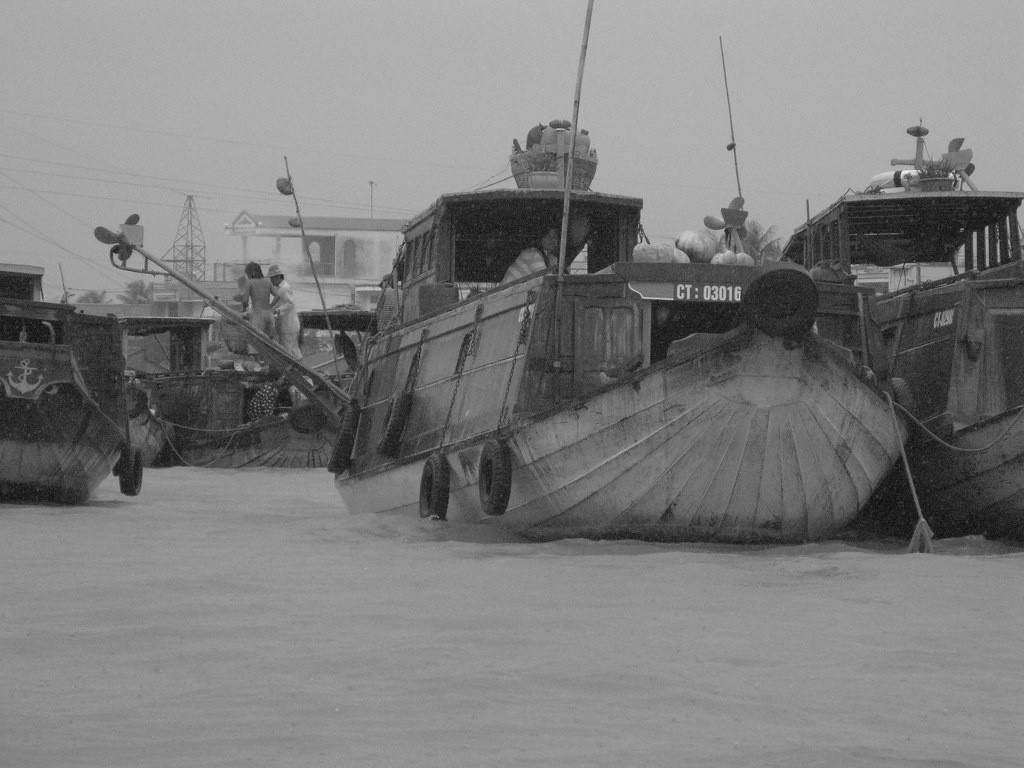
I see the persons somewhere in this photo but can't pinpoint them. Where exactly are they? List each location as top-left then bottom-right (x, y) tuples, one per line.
(498, 227), (558, 284)
(266, 265), (302, 360)
(241, 263), (280, 339)
(376, 274), (403, 333)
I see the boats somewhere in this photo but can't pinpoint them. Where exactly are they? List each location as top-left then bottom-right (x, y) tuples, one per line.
(0, 0), (1023, 550)
(323, 116), (913, 546)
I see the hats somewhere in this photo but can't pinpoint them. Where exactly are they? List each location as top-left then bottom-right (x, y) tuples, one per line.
(265, 265), (285, 277)
(378, 274), (393, 287)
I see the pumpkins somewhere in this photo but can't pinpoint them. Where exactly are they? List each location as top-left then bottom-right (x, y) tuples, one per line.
(632, 230), (755, 266)
(288, 217), (301, 227)
(276, 177), (293, 195)
